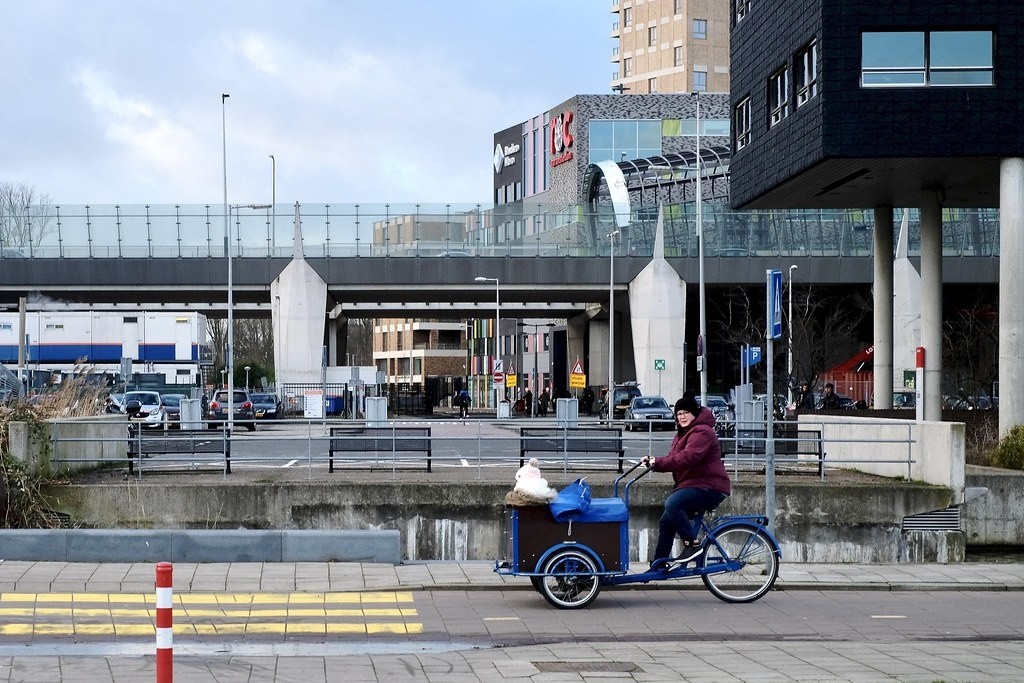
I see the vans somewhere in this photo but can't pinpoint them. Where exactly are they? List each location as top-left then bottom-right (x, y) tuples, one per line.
(868, 387), (916, 410)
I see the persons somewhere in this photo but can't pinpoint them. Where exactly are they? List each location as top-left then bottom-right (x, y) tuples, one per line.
(540, 389), (550, 417)
(458, 387), (471, 418)
(505, 458), (556, 505)
(583, 386), (595, 416)
(641, 392), (731, 572)
(823, 383), (840, 408)
(802, 384), (814, 409)
(523, 390), (533, 417)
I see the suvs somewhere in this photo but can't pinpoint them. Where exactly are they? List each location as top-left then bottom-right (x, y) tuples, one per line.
(207, 387), (257, 431)
(597, 385), (641, 424)
(625, 395), (676, 432)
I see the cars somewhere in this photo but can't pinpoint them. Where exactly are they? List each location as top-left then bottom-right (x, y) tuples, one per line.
(111, 393), (188, 427)
(942, 396), (999, 411)
(694, 391), (857, 419)
(121, 391), (168, 430)
(718, 249), (758, 257)
(249, 393), (285, 420)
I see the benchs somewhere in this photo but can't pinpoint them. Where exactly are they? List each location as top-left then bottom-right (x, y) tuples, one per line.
(519, 427), (625, 475)
(126, 428), (232, 475)
(328, 426), (434, 474)
(718, 428), (827, 476)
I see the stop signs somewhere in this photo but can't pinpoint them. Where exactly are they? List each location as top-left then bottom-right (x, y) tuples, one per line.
(493, 373), (502, 382)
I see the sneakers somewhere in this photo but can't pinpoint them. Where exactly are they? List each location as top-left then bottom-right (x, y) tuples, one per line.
(674, 544), (704, 564)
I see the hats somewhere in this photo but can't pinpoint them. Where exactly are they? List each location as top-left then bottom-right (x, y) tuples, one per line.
(675, 390), (700, 418)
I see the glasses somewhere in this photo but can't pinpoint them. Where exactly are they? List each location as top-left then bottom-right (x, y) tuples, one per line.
(677, 411), (690, 417)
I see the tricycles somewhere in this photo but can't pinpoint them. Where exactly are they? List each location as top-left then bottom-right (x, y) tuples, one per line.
(493, 458), (783, 611)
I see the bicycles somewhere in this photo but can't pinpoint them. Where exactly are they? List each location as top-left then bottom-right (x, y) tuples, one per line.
(456, 400), (473, 427)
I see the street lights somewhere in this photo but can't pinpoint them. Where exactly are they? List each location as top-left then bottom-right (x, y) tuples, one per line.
(517, 321), (556, 416)
(606, 231), (620, 428)
(649, 165), (707, 406)
(244, 366), (250, 390)
(226, 205), (273, 432)
(474, 275), (499, 419)
(789, 264), (797, 406)
